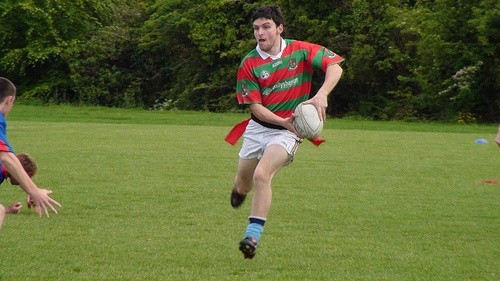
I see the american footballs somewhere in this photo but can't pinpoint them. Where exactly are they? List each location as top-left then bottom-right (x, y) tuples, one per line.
(293, 102), (323, 140)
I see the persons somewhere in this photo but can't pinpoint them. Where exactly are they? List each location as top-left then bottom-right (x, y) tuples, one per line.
(0, 152), (38, 214)
(0, 77), (62, 224)
(224, 6), (345, 259)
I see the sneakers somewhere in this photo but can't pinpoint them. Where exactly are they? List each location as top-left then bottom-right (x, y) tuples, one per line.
(239, 237), (258, 259)
(231, 189), (246, 208)
